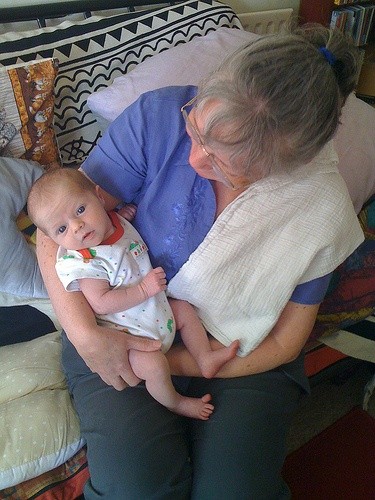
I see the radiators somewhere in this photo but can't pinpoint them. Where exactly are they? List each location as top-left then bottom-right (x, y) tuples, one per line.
(236, 9), (294, 35)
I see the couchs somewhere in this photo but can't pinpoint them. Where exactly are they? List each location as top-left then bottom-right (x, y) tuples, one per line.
(1, 0), (374, 500)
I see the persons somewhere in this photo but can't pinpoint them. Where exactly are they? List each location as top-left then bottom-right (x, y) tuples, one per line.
(27, 167), (240, 421)
(35, 22), (366, 500)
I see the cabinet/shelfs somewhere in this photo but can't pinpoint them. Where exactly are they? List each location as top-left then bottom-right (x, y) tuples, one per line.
(298, 0), (374, 28)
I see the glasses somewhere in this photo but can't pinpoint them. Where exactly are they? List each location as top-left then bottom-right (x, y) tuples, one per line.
(180, 92), (255, 191)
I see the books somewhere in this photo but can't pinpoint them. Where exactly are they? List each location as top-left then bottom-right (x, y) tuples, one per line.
(331, 0), (375, 47)
